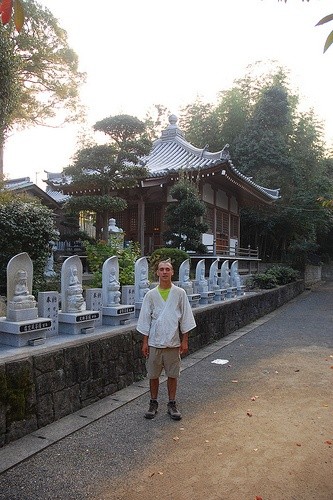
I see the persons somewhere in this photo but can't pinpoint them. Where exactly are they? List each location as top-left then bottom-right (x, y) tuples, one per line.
(182, 268), (191, 282)
(199, 266), (206, 281)
(224, 265), (229, 277)
(135, 259), (197, 420)
(67, 265), (80, 287)
(139, 267), (149, 282)
(212, 266), (219, 278)
(13, 271), (35, 303)
(234, 266), (239, 277)
(109, 267), (120, 285)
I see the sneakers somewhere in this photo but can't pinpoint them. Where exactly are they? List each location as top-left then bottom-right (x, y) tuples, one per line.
(167, 400), (182, 419)
(144, 400), (158, 419)
